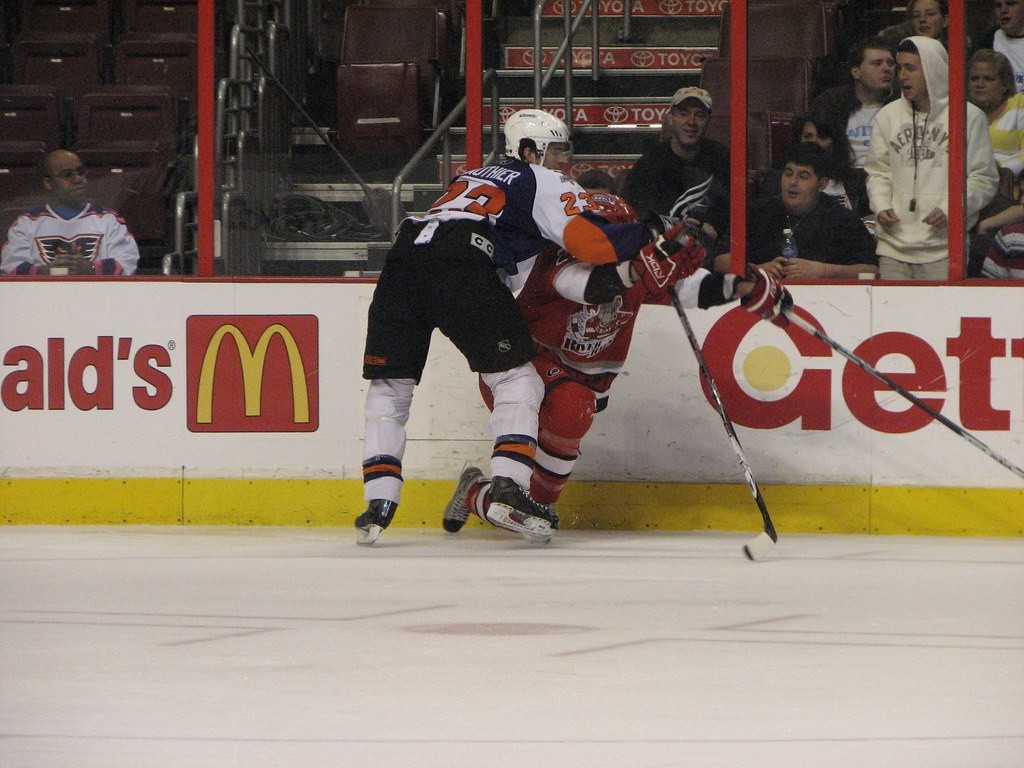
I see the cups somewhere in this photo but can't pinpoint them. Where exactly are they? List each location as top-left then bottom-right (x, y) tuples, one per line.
(858, 273), (875, 279)
(49, 268), (69, 275)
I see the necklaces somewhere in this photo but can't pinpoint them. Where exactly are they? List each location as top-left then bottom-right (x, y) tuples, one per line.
(909, 100), (930, 212)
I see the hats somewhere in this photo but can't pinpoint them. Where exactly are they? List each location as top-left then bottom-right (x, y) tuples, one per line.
(670, 87), (713, 111)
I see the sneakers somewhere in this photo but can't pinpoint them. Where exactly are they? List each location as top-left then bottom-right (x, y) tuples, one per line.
(443, 465), (491, 533)
(353, 498), (397, 545)
(487, 475), (560, 546)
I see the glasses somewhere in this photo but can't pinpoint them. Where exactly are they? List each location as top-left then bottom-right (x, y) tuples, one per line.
(47, 169), (87, 179)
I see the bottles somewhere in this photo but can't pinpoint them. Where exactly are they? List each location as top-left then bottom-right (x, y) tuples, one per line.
(781, 229), (798, 265)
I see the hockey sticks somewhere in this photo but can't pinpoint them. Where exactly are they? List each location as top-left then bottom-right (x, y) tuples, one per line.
(782, 307), (1024, 481)
(667, 285), (779, 562)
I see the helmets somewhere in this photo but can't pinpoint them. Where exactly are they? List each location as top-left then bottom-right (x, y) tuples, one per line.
(504, 109), (570, 160)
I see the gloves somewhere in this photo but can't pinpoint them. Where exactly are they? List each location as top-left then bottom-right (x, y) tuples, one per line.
(631, 222), (707, 298)
(740, 263), (795, 326)
(652, 215), (676, 233)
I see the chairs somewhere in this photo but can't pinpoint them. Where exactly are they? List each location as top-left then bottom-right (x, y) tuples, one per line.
(698, 0), (844, 191)
(0, 0), (481, 274)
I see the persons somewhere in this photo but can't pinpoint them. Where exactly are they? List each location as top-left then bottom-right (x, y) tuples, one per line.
(0, 149), (140, 277)
(620, 0), (1024, 285)
(354, 109), (680, 546)
(444, 169), (794, 532)
